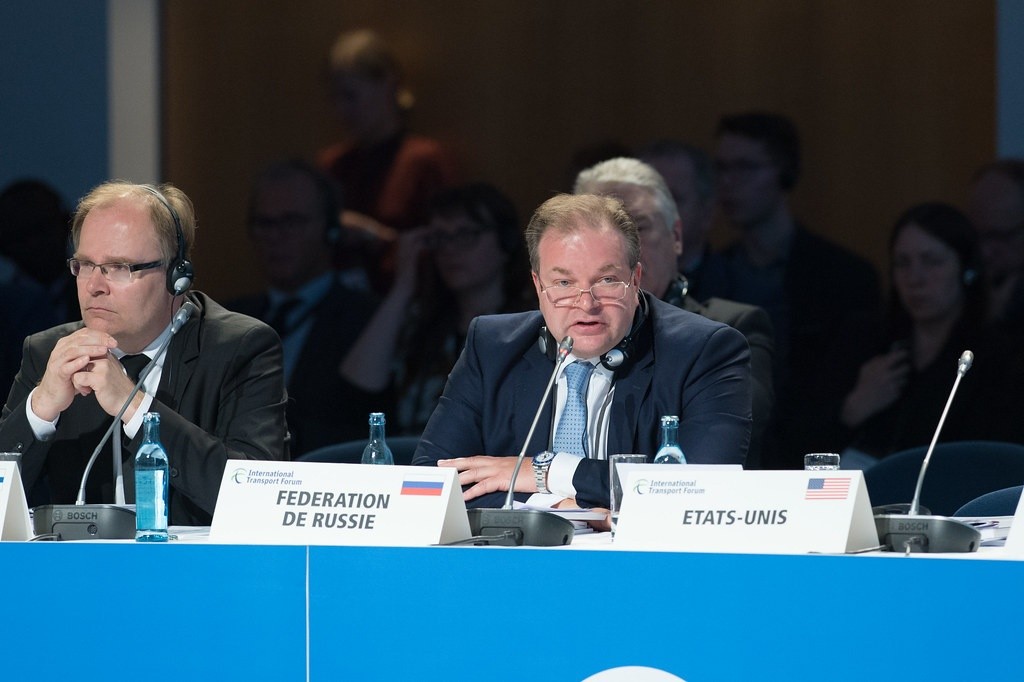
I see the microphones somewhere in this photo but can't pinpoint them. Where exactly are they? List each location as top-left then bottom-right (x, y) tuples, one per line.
(465, 336), (576, 546)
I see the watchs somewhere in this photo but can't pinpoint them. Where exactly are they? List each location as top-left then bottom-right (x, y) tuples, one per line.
(532, 451), (554, 495)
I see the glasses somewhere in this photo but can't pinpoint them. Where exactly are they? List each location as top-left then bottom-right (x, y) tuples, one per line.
(535, 268), (633, 306)
(66, 257), (171, 282)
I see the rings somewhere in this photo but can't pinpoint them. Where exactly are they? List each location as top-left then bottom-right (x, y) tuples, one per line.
(86, 365), (89, 371)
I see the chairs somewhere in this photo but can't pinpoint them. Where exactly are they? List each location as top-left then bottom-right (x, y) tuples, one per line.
(864, 440), (1024, 516)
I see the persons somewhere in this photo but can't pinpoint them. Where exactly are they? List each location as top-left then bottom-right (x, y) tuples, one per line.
(224, 27), (540, 463)
(0, 174), (81, 406)
(780, 158), (1024, 470)
(572, 110), (910, 468)
(0, 182), (286, 526)
(412, 194), (753, 532)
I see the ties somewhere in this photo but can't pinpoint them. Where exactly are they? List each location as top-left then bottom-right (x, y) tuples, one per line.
(119, 353), (152, 505)
(553, 361), (596, 460)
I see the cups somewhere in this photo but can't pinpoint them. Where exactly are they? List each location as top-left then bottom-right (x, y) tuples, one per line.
(805, 453), (840, 470)
(609, 454), (648, 543)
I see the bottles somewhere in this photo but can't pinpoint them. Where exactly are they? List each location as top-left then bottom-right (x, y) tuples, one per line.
(654, 416), (687, 464)
(134, 412), (169, 542)
(362, 413), (394, 465)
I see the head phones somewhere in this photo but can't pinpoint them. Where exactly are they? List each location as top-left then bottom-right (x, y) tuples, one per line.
(962, 259), (983, 288)
(539, 289), (650, 372)
(140, 184), (193, 298)
(666, 272), (689, 308)
(874, 350), (978, 554)
(32, 302), (198, 539)
